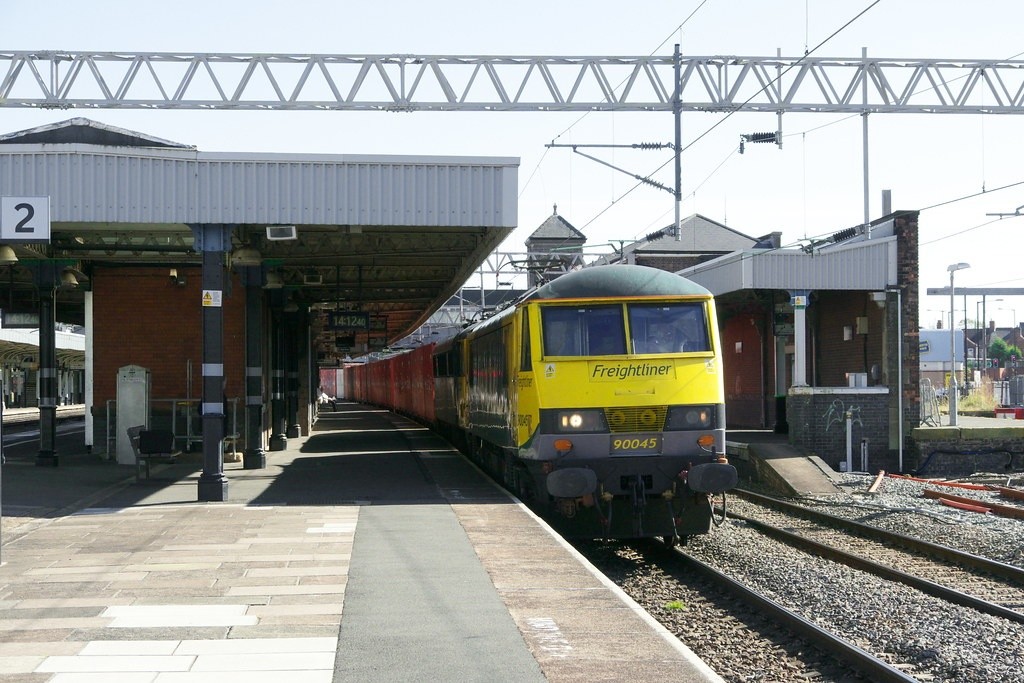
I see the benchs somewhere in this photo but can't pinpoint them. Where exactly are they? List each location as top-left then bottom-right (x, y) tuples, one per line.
(126, 423), (182, 483)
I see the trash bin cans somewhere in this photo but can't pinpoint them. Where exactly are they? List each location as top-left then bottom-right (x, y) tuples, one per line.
(994, 408), (1024, 419)
(774, 397), (789, 434)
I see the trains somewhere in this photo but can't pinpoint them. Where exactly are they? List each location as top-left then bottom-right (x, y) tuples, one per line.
(319, 264), (739, 548)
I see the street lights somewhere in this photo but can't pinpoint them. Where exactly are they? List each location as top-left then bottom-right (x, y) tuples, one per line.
(927, 309), (945, 329)
(999, 307), (1017, 354)
(947, 263), (970, 426)
(948, 310), (965, 329)
(977, 299), (1004, 370)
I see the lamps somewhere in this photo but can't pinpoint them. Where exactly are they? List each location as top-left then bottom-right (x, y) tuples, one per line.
(0, 246), (18, 266)
(59, 270), (80, 290)
(75, 237), (87, 245)
(169, 267), (177, 281)
(259, 265), (284, 290)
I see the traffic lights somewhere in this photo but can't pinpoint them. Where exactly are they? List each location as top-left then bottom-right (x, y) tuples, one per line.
(993, 358), (998, 367)
(1012, 355), (1016, 363)
